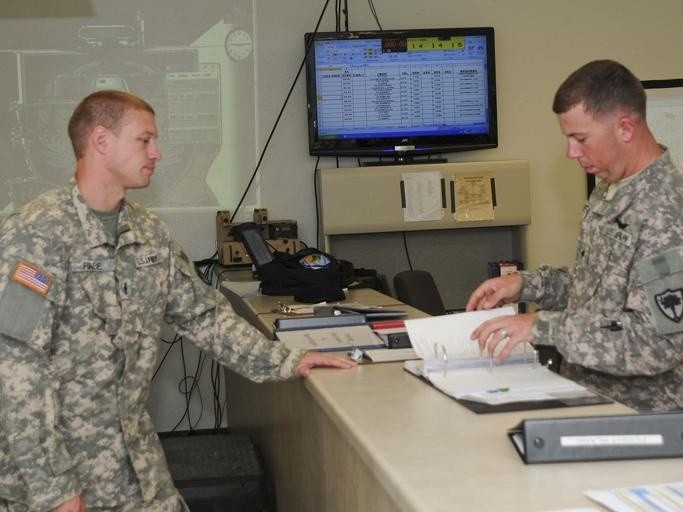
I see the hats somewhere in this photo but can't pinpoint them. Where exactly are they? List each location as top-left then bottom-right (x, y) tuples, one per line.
(293, 248), (345, 302)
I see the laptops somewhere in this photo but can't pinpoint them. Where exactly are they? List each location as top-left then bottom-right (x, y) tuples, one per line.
(239, 221), (283, 273)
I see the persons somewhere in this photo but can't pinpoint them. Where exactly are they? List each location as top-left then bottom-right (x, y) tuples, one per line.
(466, 60), (683, 414)
(1, 90), (358, 512)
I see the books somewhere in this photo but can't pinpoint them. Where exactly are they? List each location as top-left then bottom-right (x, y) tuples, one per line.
(274, 313), (370, 330)
(335, 304), (409, 322)
(402, 302), (614, 417)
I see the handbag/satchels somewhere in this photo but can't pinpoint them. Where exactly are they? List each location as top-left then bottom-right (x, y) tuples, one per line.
(257, 251), (355, 295)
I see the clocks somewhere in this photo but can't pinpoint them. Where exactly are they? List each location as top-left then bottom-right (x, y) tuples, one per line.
(224, 27), (253, 64)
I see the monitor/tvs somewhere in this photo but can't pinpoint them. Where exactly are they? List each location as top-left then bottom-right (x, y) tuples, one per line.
(303, 25), (499, 167)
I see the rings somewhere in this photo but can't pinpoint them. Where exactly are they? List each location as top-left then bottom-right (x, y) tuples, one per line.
(501, 329), (512, 337)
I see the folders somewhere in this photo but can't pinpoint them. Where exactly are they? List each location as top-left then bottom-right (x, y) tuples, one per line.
(507, 409), (683, 464)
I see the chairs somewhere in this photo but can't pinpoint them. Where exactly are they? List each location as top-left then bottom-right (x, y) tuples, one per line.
(394, 267), (466, 316)
(153, 425), (262, 510)
